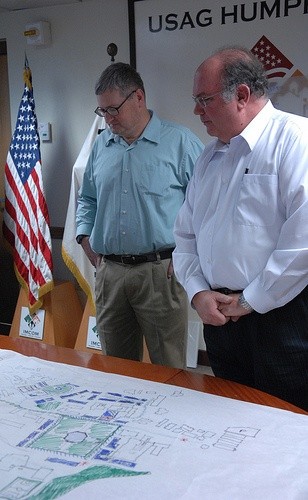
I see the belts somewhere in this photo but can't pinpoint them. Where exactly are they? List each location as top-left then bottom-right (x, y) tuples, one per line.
(99, 248), (173, 265)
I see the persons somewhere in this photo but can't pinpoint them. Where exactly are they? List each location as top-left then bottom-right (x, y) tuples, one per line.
(74, 64), (205, 371)
(172, 45), (308, 416)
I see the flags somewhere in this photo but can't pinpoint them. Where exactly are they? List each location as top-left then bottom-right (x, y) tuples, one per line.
(4, 56), (56, 314)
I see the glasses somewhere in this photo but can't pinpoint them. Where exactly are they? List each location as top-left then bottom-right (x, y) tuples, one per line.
(94, 90), (138, 118)
(191, 85), (236, 108)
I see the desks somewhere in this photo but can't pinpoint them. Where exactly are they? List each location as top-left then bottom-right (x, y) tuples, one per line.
(0, 334), (308, 416)
